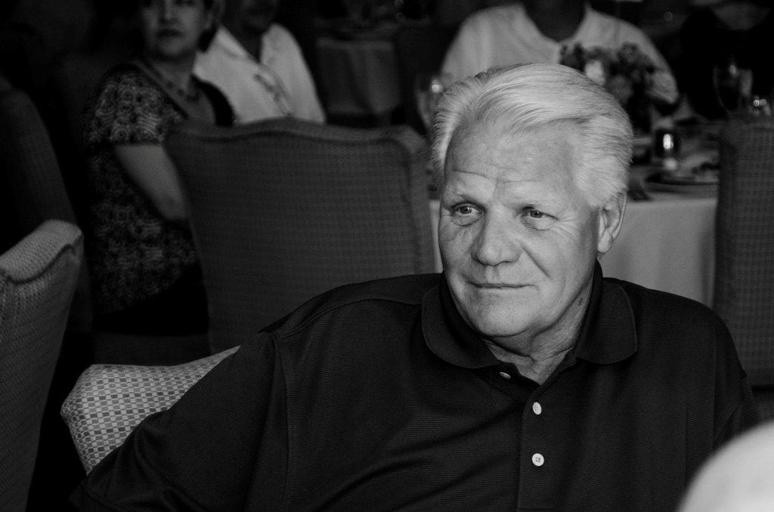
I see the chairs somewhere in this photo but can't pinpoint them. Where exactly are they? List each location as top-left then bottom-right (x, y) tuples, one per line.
(1, 1), (774, 512)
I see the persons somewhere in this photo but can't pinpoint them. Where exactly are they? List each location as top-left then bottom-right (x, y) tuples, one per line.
(68, 58), (760, 509)
(192, 1), (325, 129)
(434, 0), (689, 161)
(83, 0), (232, 331)
